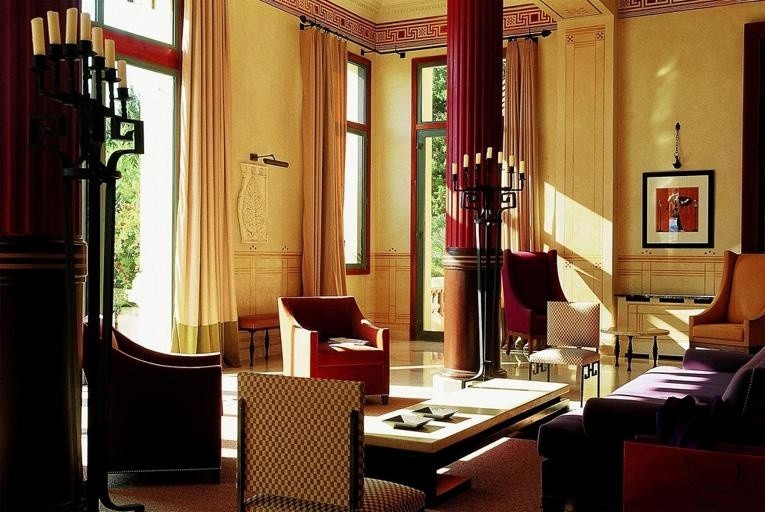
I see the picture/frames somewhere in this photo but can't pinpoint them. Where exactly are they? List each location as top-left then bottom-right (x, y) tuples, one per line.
(641, 170), (714, 248)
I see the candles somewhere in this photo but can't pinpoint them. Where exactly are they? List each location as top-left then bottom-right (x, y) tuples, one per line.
(30, 7), (136, 101)
(450, 147), (526, 182)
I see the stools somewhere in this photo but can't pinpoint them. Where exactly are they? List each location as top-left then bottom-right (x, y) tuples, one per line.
(237, 312), (281, 368)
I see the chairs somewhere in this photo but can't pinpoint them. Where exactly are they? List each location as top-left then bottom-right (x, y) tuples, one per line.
(82, 320), (222, 483)
(502, 249), (581, 358)
(528, 301), (599, 409)
(689, 249), (765, 354)
(277, 295), (390, 405)
(236, 371), (425, 512)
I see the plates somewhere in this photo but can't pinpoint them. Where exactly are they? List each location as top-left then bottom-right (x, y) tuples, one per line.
(412, 406), (461, 420)
(383, 413), (433, 429)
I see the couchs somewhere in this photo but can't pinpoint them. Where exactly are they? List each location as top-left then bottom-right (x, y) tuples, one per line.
(537, 346), (765, 512)
